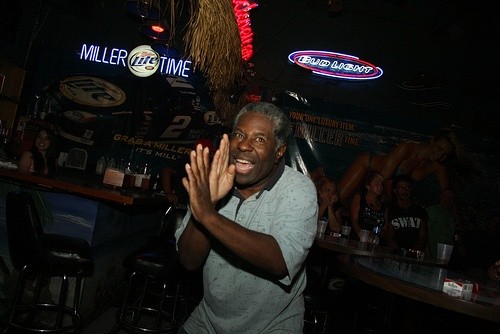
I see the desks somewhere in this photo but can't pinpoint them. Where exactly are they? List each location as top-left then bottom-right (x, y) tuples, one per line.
(314, 234), (500, 334)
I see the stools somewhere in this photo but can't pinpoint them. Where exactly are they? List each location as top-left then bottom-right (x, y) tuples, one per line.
(105, 246), (192, 334)
(2, 191), (94, 334)
(303, 279), (420, 334)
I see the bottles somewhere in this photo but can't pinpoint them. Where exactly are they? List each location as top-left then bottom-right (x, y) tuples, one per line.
(340, 204), (352, 238)
(95, 155), (160, 192)
(27, 86), (53, 119)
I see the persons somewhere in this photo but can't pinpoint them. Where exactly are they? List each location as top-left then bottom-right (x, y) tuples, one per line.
(18, 128), (55, 175)
(309, 134), (455, 201)
(315, 176), (342, 232)
(425, 188), (471, 268)
(175, 99), (319, 334)
(350, 172), (385, 248)
(385, 174), (431, 259)
(135, 143), (178, 204)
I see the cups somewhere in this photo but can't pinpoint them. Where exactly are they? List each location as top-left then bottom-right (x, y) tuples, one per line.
(436, 243), (454, 265)
(317, 220), (329, 234)
(360, 229), (370, 242)
